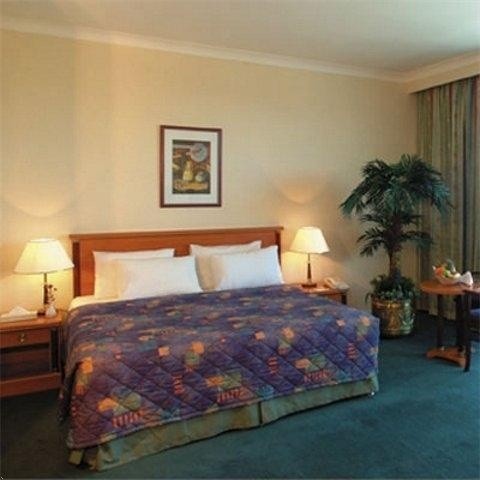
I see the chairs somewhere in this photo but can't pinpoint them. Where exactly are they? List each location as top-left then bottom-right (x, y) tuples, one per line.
(462, 288), (480, 373)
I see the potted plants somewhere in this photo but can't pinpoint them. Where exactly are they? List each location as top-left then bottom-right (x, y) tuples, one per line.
(337, 153), (457, 338)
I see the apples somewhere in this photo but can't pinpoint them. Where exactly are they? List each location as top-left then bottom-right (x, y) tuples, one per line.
(444, 270), (450, 277)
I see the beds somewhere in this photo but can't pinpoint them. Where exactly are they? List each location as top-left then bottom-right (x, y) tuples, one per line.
(54, 225), (381, 474)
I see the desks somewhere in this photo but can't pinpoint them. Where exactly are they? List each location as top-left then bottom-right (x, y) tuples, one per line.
(419, 278), (480, 368)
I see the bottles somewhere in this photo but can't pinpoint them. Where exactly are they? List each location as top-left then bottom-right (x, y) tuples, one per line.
(45, 284), (57, 318)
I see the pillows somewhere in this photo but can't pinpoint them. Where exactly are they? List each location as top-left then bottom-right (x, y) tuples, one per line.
(90, 239), (284, 301)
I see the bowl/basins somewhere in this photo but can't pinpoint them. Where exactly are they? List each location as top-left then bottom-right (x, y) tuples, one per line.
(435, 275), (460, 286)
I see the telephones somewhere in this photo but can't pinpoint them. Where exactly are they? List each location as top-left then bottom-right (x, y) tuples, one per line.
(324, 276), (350, 289)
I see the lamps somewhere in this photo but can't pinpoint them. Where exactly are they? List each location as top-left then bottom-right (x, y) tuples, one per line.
(13, 239), (76, 315)
(288, 227), (330, 287)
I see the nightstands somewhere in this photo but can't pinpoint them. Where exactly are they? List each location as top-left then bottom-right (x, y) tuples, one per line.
(291, 283), (347, 305)
(0, 307), (69, 422)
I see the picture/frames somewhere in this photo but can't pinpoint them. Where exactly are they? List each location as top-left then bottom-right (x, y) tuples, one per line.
(160, 124), (222, 208)
